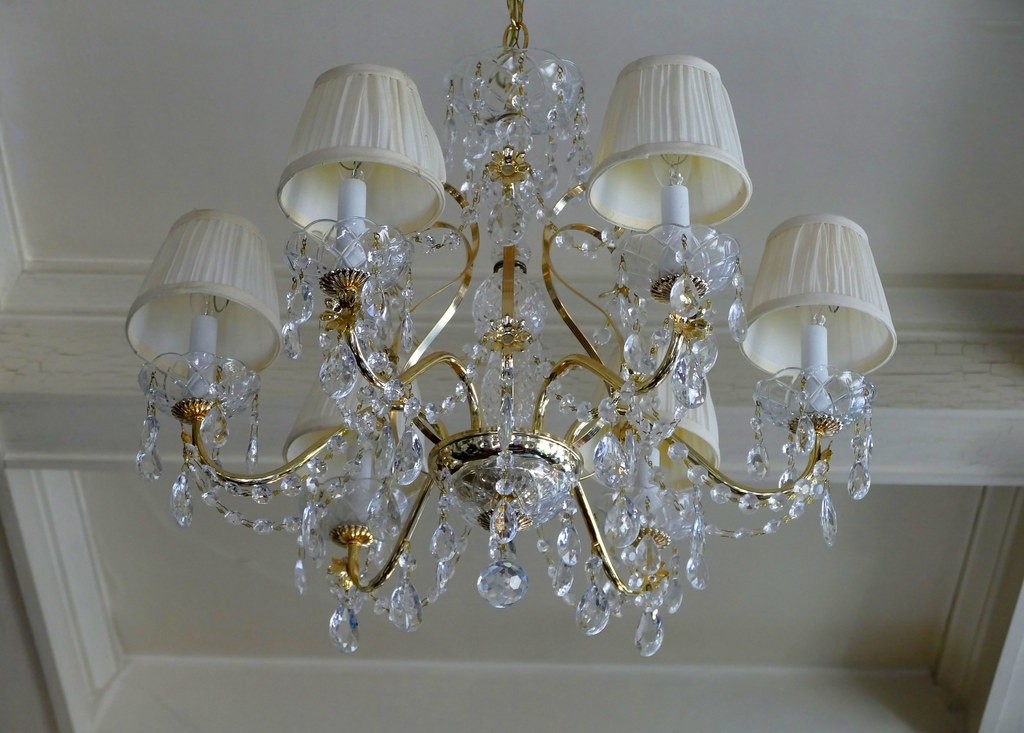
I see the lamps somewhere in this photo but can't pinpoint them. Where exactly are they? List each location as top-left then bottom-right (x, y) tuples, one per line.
(117, 0), (905, 658)
